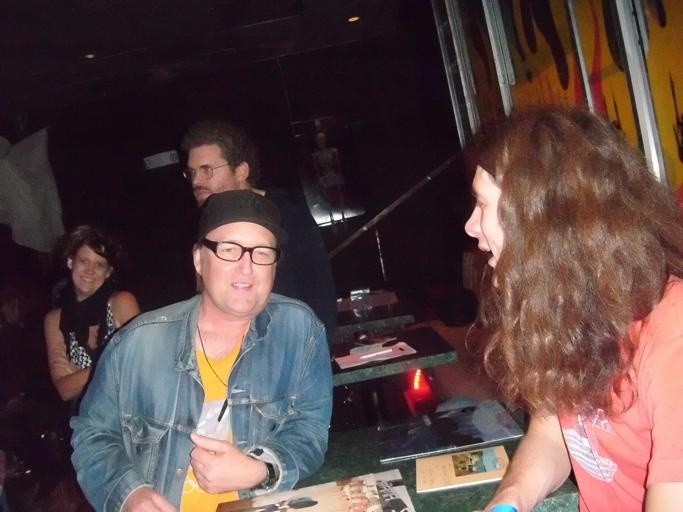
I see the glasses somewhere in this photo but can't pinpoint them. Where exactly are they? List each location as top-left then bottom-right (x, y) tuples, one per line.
(182, 162), (230, 180)
(202, 239), (282, 266)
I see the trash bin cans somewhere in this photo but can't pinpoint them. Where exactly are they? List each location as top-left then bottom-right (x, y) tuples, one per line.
(441, 288), (474, 327)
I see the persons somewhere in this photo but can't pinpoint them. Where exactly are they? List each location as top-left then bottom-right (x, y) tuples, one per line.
(463, 100), (683, 511)
(41, 224), (142, 511)
(180, 119), (339, 356)
(66, 189), (334, 511)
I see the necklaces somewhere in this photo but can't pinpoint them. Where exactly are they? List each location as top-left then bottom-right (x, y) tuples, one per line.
(196, 324), (229, 387)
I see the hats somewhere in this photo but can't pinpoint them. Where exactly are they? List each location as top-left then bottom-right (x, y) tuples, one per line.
(193, 189), (290, 250)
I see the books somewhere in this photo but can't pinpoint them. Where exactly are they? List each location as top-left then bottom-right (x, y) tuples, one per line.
(378, 398), (523, 463)
(213, 467), (417, 511)
(416, 443), (511, 494)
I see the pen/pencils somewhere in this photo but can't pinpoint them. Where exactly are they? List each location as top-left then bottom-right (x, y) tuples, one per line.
(360, 348), (393, 359)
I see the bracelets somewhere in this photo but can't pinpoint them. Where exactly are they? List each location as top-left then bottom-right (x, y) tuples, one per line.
(261, 461), (275, 491)
